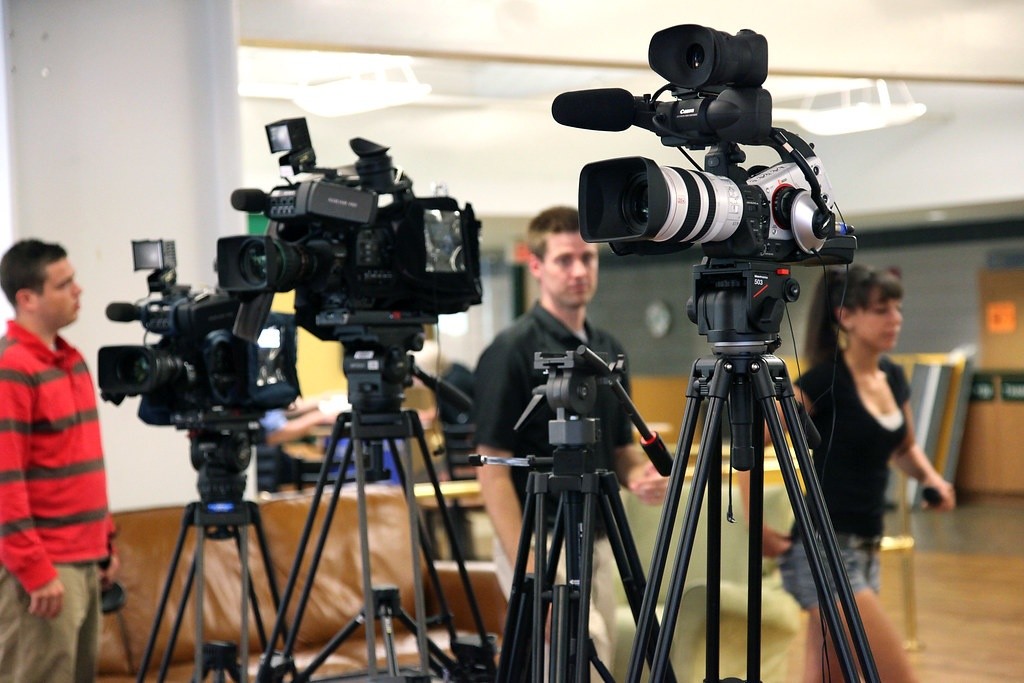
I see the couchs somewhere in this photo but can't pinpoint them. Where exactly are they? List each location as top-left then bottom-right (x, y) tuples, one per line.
(94, 481), (517, 682)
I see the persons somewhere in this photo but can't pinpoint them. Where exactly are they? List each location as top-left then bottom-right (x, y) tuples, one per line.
(0, 239), (122, 683)
(247, 333), (479, 497)
(467, 204), (676, 683)
(736, 263), (960, 683)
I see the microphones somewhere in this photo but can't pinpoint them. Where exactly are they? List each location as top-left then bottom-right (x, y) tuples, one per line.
(923, 487), (943, 506)
(107, 303), (140, 321)
(230, 189), (271, 213)
(552, 88), (637, 131)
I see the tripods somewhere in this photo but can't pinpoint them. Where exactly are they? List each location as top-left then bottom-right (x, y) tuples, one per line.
(136, 235), (881, 683)
